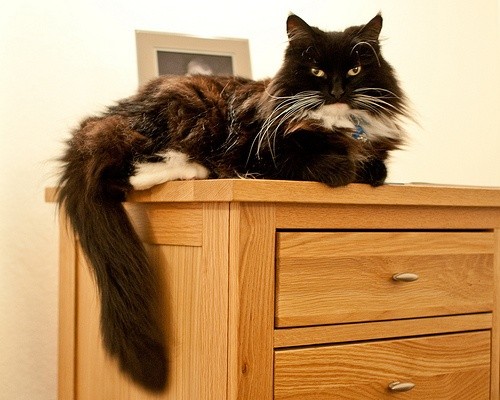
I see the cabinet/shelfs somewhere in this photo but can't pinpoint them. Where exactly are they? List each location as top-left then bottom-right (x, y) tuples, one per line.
(43, 176), (500, 400)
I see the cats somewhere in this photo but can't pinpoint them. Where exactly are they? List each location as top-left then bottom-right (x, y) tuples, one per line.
(52, 11), (424, 395)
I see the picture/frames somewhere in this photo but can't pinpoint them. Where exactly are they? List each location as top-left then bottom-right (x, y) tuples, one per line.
(134, 28), (253, 95)
